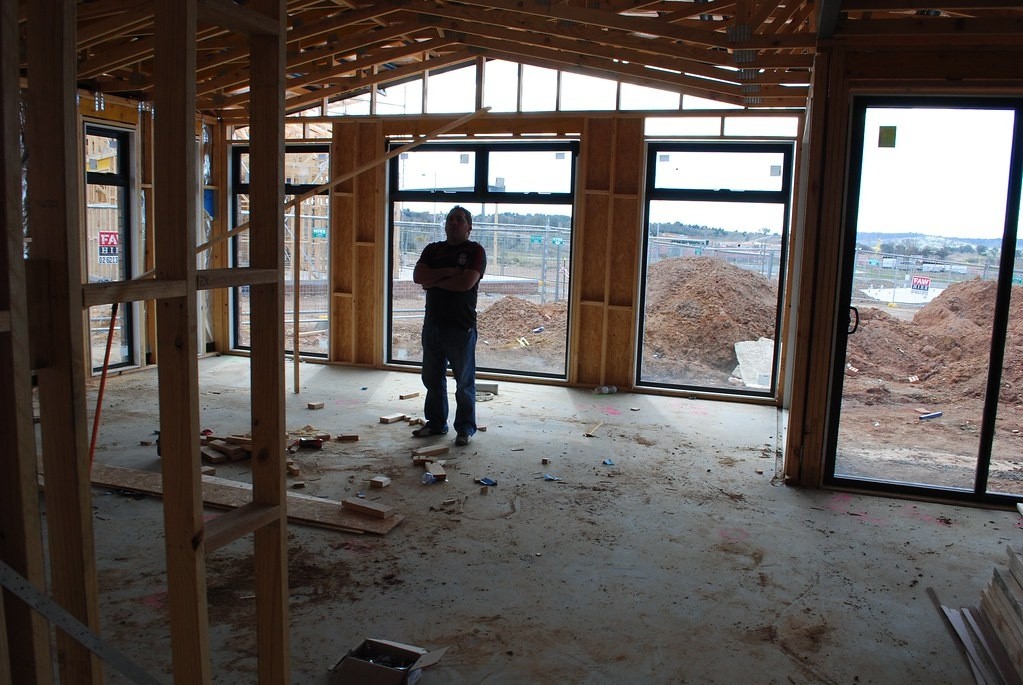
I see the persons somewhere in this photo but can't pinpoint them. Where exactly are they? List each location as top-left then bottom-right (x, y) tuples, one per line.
(411, 206), (487, 447)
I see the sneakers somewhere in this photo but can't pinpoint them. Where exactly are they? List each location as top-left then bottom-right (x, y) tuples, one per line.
(412, 422), (449, 437)
(456, 433), (471, 445)
